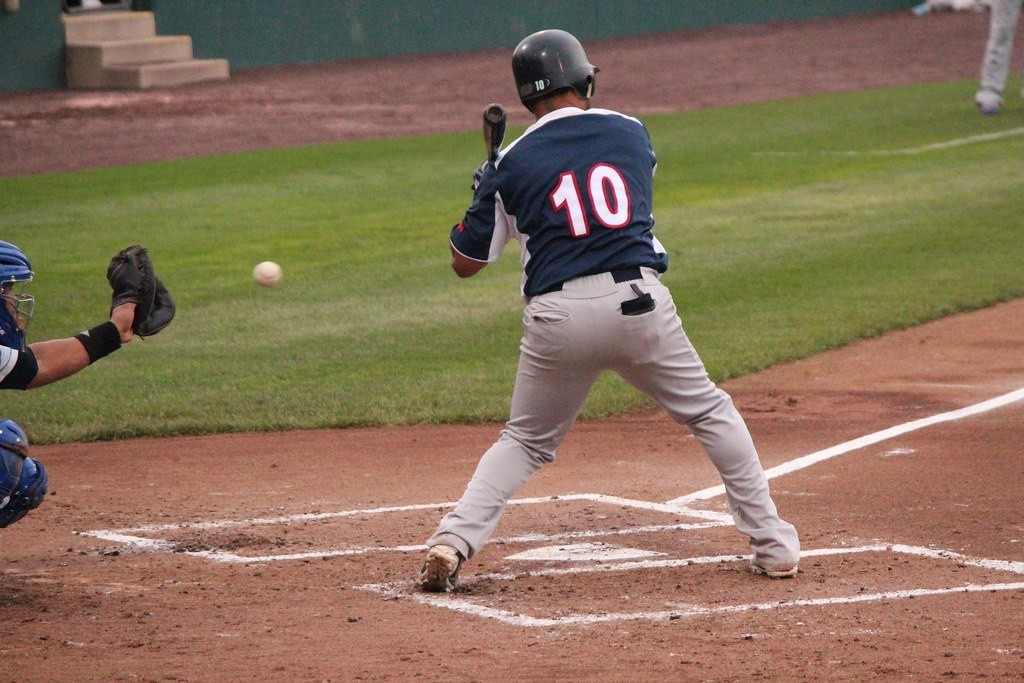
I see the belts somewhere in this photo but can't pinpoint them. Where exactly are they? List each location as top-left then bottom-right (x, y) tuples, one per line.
(537, 266), (643, 294)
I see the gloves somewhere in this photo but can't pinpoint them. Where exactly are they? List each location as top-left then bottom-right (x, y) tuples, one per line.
(470, 160), (489, 189)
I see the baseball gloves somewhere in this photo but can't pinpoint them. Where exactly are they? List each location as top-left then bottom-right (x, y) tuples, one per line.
(107, 243), (176, 337)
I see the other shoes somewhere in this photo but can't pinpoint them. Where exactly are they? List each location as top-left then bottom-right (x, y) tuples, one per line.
(419, 545), (465, 593)
(750, 556), (800, 580)
(975, 88), (1003, 113)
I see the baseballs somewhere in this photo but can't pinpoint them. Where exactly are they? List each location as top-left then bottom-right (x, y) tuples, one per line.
(253, 262), (283, 289)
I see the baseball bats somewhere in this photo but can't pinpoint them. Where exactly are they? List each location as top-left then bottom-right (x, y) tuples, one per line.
(482, 104), (507, 163)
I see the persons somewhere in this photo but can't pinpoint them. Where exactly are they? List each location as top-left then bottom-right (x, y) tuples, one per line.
(421, 29), (800, 593)
(925, 0), (1023, 112)
(0, 240), (175, 528)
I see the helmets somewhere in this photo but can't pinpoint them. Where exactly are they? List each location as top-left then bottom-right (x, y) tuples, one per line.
(0, 240), (34, 353)
(512, 29), (599, 113)
